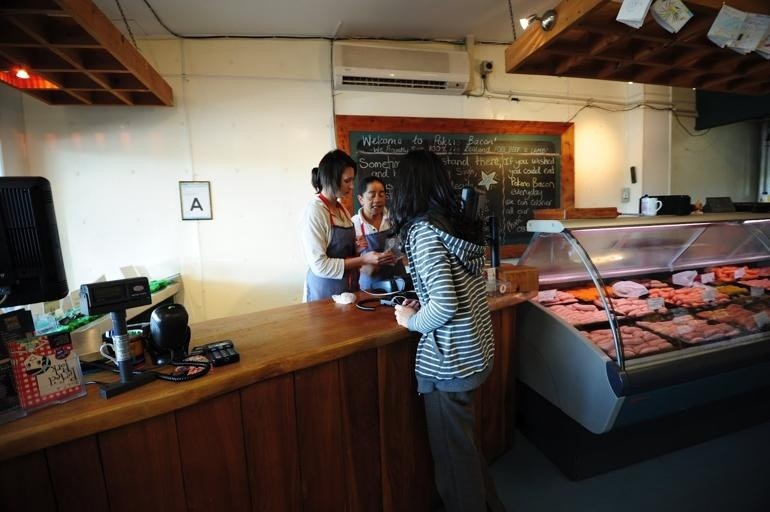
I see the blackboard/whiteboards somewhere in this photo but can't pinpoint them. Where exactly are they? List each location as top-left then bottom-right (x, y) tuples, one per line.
(334, 114), (574, 260)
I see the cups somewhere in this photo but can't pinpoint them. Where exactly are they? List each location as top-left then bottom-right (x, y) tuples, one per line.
(640, 198), (662, 217)
(99, 329), (146, 369)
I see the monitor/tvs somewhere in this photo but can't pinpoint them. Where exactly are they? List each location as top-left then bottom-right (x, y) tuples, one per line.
(460, 187), (479, 219)
(0, 176), (69, 309)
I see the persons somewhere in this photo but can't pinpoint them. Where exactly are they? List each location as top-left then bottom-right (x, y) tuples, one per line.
(350, 175), (407, 290)
(392, 150), (503, 512)
(303, 149), (395, 300)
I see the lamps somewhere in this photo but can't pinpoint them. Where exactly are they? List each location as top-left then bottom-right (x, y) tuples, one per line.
(518, 9), (557, 33)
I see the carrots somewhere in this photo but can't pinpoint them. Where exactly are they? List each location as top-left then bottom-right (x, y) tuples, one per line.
(704, 266), (760, 283)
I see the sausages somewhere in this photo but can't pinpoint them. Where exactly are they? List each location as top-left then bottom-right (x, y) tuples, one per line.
(531, 278), (761, 360)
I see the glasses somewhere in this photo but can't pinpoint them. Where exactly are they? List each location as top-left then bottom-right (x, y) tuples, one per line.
(390, 295), (420, 310)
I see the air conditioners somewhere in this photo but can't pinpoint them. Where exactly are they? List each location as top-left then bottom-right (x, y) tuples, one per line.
(330, 38), (472, 97)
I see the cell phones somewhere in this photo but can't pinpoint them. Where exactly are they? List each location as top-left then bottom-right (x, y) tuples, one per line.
(394, 297), (408, 306)
